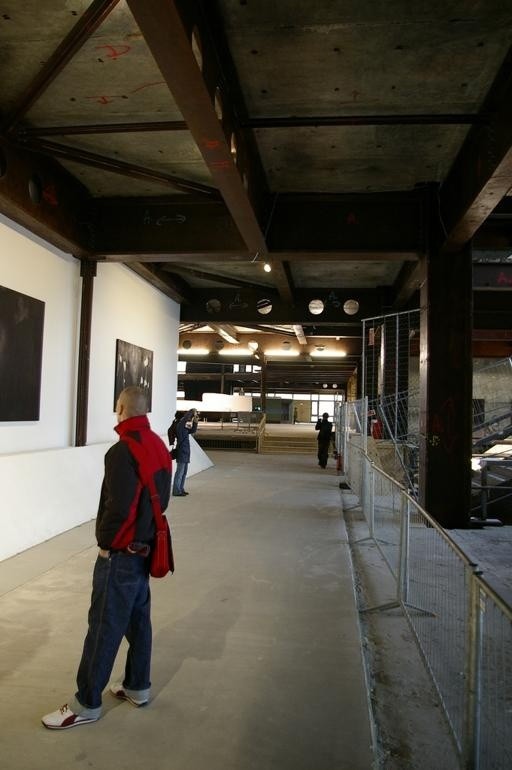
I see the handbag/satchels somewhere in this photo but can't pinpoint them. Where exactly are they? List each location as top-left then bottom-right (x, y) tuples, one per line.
(169, 448), (183, 460)
(149, 515), (174, 578)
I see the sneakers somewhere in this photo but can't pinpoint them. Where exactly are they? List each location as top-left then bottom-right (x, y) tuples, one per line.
(178, 491), (188, 496)
(109, 681), (148, 707)
(41, 703), (98, 730)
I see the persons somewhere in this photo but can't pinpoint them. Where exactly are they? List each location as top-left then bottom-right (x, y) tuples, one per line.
(172, 407), (199, 497)
(40, 385), (172, 730)
(293, 407), (300, 424)
(116, 353), (152, 412)
(314, 412), (333, 470)
